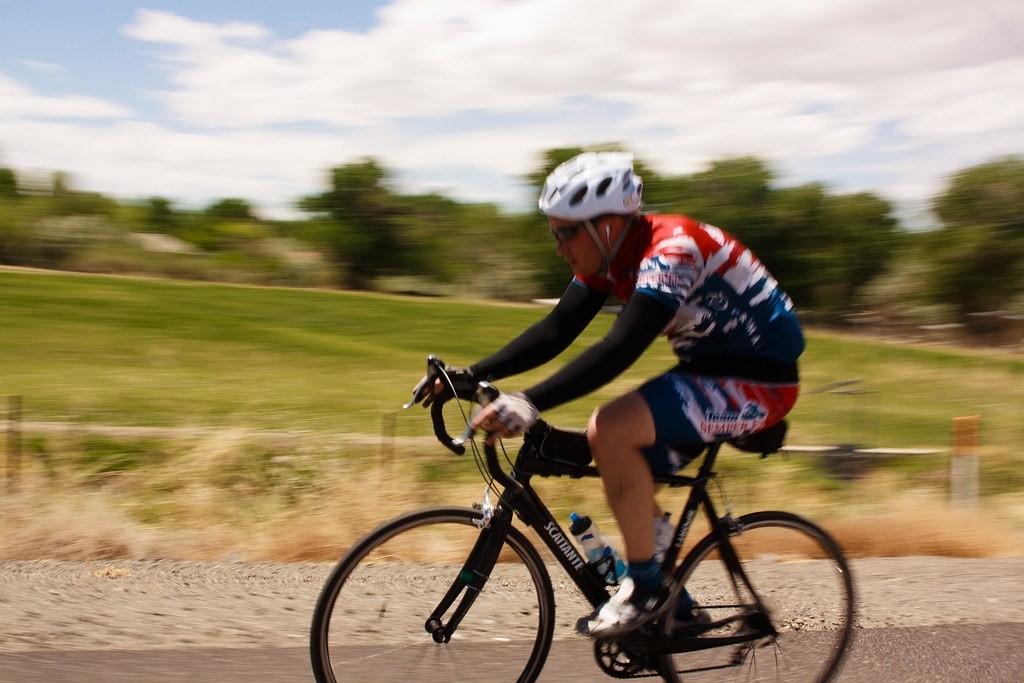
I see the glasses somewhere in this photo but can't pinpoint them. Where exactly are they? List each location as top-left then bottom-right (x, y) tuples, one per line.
(547, 215), (607, 244)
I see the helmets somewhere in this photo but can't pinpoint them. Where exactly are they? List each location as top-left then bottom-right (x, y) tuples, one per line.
(537, 148), (643, 219)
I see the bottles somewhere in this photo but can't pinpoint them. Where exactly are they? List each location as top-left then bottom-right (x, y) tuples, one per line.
(569, 512), (627, 584)
(649, 510), (678, 572)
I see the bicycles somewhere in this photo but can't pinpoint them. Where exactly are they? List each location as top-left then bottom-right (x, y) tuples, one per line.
(311, 355), (858, 683)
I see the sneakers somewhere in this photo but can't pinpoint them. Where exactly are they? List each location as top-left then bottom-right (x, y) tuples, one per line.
(641, 600), (711, 645)
(576, 569), (680, 638)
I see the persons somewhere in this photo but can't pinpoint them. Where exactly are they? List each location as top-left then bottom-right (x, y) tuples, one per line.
(410, 150), (806, 642)
